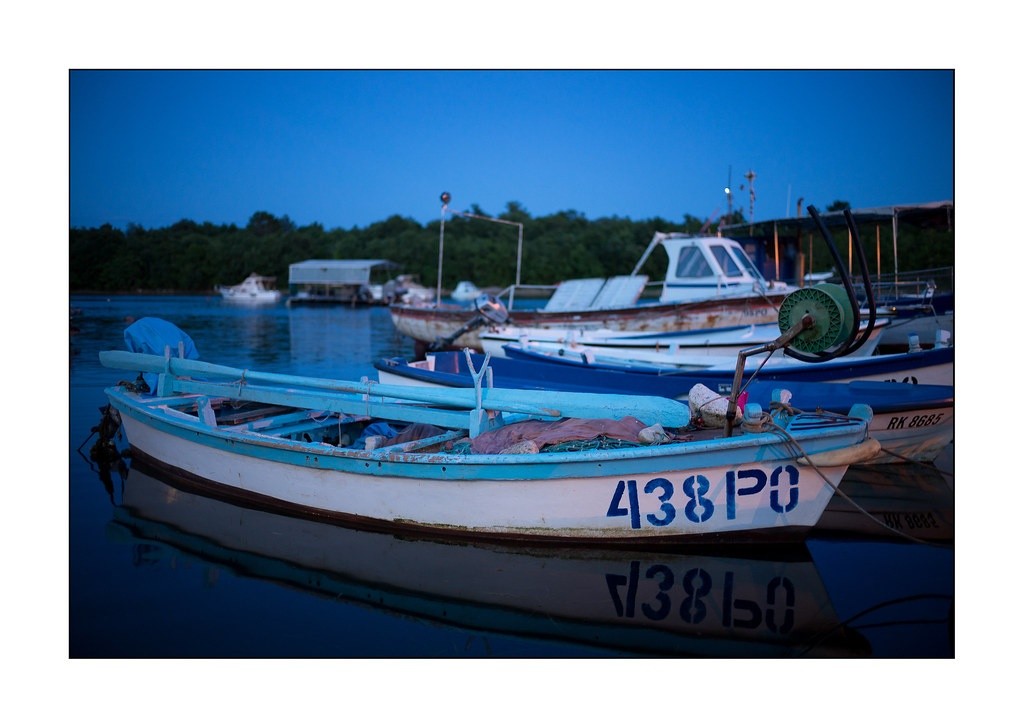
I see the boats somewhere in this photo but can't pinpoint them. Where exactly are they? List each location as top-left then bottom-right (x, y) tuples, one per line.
(98, 204), (878, 552)
(219, 273), (281, 304)
(107, 461), (858, 656)
(373, 230), (955, 465)
